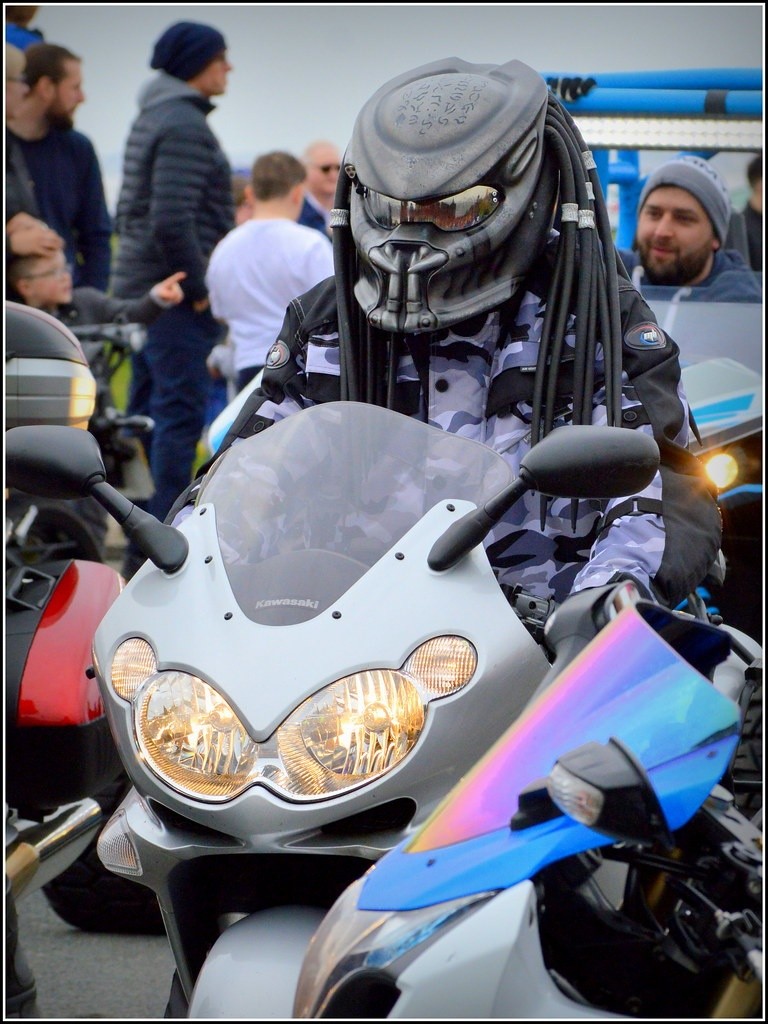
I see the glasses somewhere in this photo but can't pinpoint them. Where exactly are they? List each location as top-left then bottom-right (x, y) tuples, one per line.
(309, 164), (340, 173)
(7, 73), (27, 85)
(17, 262), (72, 280)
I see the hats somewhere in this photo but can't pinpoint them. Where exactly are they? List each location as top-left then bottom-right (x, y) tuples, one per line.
(637, 154), (731, 252)
(149, 20), (226, 82)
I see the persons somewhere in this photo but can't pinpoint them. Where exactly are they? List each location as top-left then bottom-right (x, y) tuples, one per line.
(725, 153), (762, 271)
(205, 176), (253, 427)
(294, 139), (341, 243)
(6, 42), (68, 259)
(204, 150), (336, 394)
(110, 22), (237, 579)
(193, 57), (724, 665)
(6, 6), (45, 52)
(22, 41), (113, 294)
(614, 156), (762, 304)
(5, 246), (188, 559)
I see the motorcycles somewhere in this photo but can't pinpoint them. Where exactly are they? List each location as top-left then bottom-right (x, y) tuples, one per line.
(5, 301), (763, 1017)
